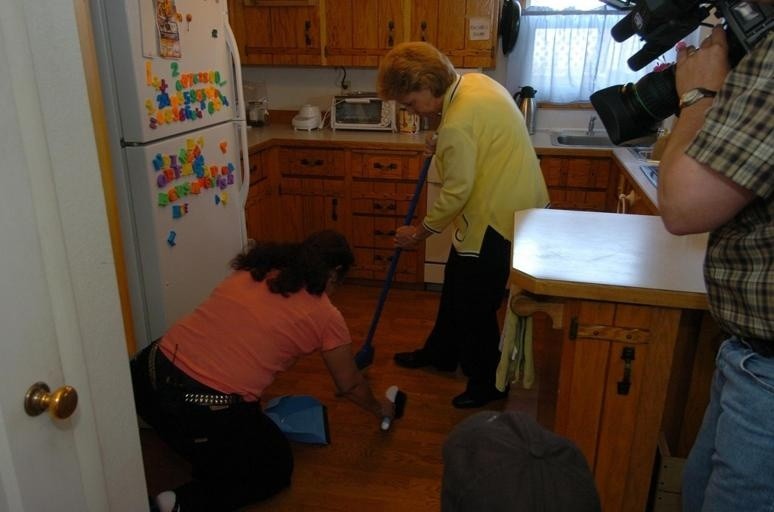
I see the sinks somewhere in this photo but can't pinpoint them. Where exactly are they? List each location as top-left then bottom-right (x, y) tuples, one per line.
(550, 131), (622, 147)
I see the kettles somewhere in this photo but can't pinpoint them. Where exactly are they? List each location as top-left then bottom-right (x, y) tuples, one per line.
(513, 86), (539, 135)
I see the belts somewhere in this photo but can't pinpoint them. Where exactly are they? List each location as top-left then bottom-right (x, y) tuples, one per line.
(149, 340), (243, 408)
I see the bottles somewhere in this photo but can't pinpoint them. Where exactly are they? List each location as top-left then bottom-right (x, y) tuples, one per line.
(248, 100), (266, 127)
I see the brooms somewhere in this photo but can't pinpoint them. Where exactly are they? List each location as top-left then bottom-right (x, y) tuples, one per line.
(338, 151), (433, 421)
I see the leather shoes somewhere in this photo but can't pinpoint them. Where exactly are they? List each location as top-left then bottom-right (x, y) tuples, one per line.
(394, 350), (458, 373)
(452, 383), (510, 409)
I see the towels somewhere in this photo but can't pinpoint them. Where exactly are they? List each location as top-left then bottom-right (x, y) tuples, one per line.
(490, 281), (537, 394)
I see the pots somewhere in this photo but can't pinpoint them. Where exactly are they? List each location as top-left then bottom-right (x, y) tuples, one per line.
(502, 0), (522, 54)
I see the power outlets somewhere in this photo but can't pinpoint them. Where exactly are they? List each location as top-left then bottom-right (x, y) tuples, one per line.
(342, 79), (351, 89)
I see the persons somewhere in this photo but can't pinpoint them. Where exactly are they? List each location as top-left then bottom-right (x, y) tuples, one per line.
(377, 41), (549, 408)
(658, 23), (774, 512)
(130, 230), (397, 512)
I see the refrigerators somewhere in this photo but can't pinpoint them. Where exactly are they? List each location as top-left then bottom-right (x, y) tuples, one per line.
(87, 1), (252, 357)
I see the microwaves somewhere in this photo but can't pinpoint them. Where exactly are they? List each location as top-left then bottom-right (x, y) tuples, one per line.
(330, 93), (398, 134)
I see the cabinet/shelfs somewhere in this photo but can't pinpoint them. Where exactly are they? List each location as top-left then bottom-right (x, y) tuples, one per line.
(241, 149), (272, 247)
(349, 148), (423, 287)
(228, 0), (323, 68)
(323, 0), (496, 69)
(538, 153), (615, 213)
(272, 145), (348, 244)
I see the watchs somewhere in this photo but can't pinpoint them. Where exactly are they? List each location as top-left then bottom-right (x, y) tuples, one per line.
(678, 88), (717, 109)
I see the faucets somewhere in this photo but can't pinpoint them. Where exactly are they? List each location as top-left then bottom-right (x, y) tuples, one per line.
(585, 116), (597, 136)
